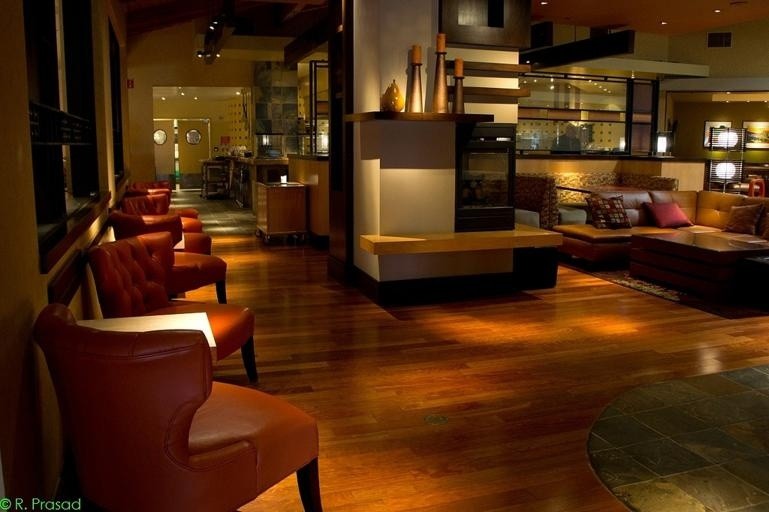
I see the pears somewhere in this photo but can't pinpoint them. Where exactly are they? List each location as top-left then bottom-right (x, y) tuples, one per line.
(386, 79), (405, 112)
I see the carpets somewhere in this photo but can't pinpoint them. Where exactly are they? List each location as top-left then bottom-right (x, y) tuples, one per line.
(586, 363), (769, 512)
(587, 271), (688, 304)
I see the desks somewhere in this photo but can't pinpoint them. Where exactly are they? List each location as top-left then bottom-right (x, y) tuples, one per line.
(628, 233), (769, 302)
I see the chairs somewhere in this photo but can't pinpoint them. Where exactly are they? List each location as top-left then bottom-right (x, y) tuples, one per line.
(31, 180), (322, 511)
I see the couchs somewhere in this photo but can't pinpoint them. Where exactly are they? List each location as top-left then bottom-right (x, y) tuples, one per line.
(515, 172), (769, 272)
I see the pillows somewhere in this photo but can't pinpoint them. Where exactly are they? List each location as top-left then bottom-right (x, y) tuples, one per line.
(585, 195), (763, 235)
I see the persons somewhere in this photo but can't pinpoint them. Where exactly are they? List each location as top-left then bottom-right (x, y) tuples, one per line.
(550, 124), (581, 154)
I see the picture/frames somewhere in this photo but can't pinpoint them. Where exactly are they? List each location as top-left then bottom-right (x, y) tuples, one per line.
(703, 120), (769, 150)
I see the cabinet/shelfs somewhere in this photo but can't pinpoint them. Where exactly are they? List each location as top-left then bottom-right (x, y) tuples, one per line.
(445, 60), (531, 96)
